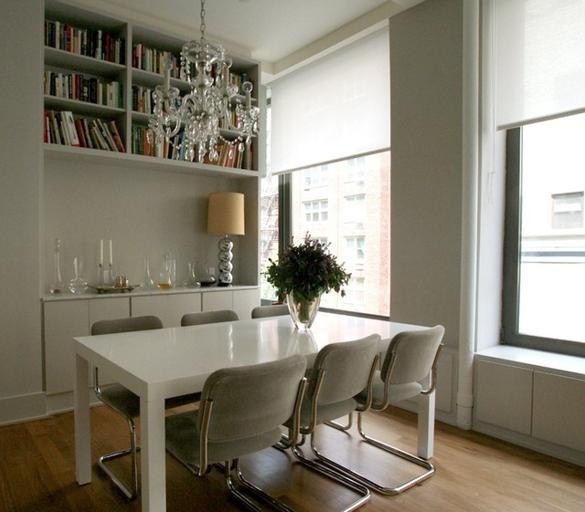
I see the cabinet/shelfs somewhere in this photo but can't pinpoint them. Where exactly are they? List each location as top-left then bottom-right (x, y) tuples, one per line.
(131, 291), (203, 329)
(473, 354), (585, 453)
(42, 0), (268, 178)
(203, 287), (261, 321)
(40, 296), (132, 418)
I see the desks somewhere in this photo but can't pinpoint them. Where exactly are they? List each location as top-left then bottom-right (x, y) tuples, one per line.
(70, 309), (438, 512)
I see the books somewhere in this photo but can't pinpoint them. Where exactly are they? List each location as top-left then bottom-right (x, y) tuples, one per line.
(44, 19), (255, 169)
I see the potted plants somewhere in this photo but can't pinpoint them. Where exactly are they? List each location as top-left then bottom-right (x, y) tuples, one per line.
(260, 227), (353, 333)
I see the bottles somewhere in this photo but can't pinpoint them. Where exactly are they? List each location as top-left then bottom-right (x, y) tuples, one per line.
(138, 256), (155, 292)
(157, 255), (171, 289)
(182, 263), (201, 288)
(68, 256), (88, 296)
(47, 239), (66, 295)
(200, 264), (216, 286)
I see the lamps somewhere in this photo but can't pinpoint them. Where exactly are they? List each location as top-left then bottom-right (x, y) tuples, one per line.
(206, 191), (247, 286)
(142, 0), (263, 165)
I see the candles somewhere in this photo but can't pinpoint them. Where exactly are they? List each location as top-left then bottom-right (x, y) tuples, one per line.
(108, 240), (113, 264)
(99, 239), (105, 265)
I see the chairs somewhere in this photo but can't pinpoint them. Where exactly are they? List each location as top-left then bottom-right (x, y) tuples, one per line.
(181, 308), (239, 326)
(91, 315), (203, 503)
(350, 321), (444, 413)
(164, 353), (371, 512)
(252, 303), (292, 318)
(281, 333), (434, 495)
